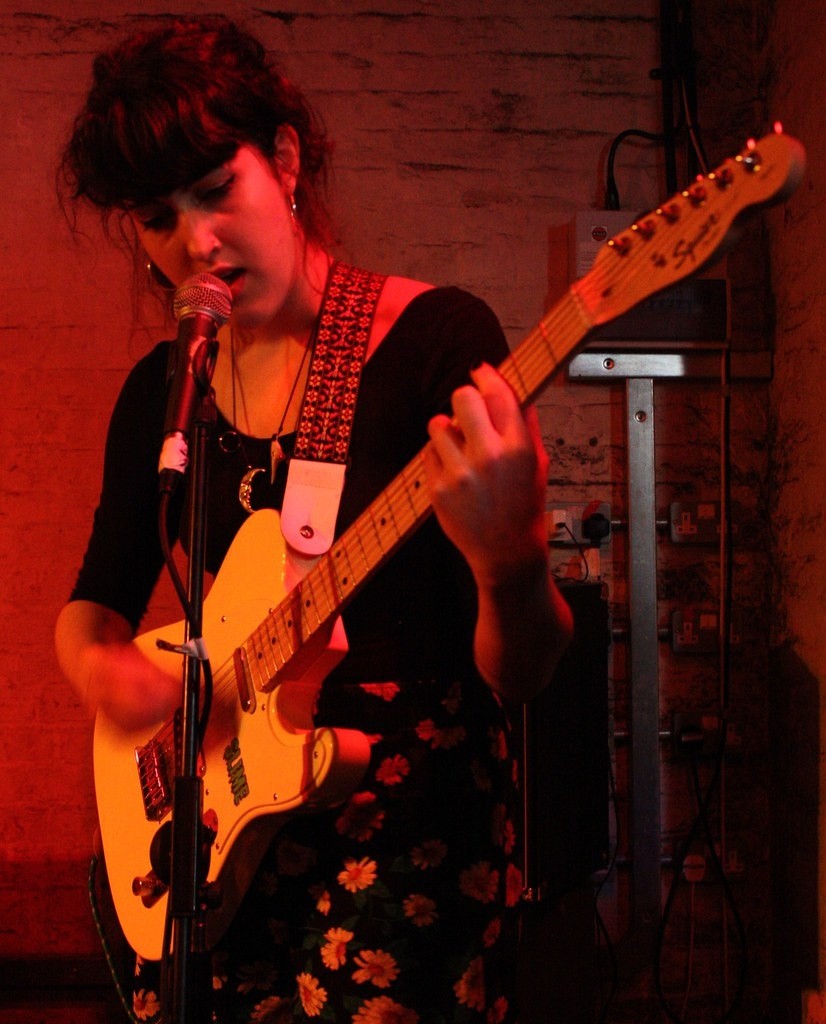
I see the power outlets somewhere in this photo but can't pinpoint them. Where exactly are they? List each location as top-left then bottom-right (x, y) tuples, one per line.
(669, 495), (751, 544)
(670, 607), (751, 657)
(545, 502), (612, 546)
(674, 829), (758, 885)
(671, 710), (754, 759)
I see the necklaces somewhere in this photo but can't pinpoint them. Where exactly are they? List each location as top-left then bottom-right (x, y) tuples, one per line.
(219, 260), (338, 512)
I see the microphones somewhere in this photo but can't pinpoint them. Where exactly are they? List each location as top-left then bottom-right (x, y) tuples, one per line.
(156, 272), (232, 495)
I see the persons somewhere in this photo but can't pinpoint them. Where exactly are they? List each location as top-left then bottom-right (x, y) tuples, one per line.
(53, 17), (576, 1024)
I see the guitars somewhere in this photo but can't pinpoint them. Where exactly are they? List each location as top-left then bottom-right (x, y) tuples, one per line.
(89, 113), (808, 967)
